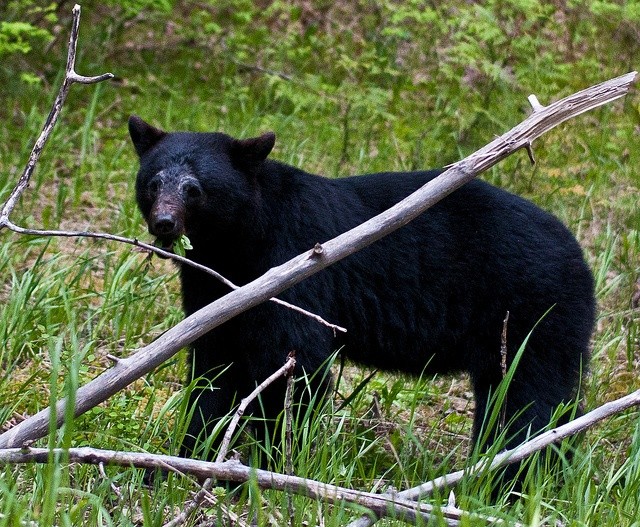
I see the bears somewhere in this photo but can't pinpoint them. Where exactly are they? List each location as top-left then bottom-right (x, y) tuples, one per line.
(128, 115), (596, 507)
